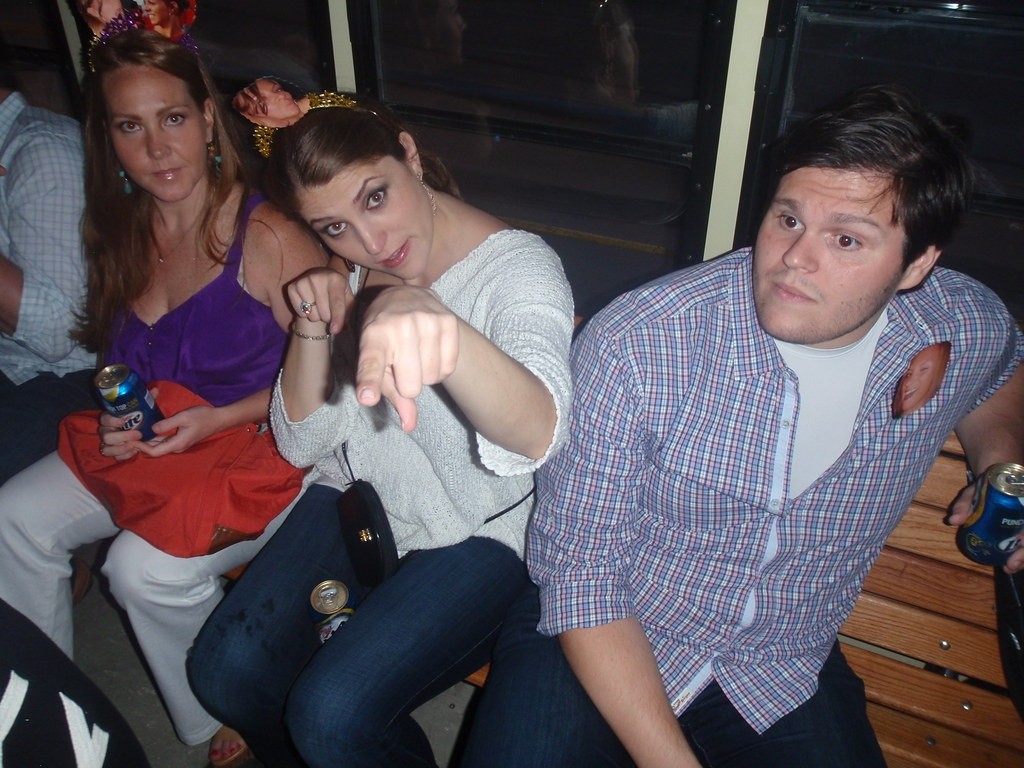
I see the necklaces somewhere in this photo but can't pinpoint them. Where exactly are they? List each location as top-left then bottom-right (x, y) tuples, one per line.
(147, 209), (201, 262)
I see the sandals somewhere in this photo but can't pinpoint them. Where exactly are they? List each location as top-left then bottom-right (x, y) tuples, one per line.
(209, 726), (248, 766)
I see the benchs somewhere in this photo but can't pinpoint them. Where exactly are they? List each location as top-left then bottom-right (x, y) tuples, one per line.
(223, 318), (1024, 768)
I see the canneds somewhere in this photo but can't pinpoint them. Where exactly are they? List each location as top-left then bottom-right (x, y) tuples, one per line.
(307, 579), (359, 643)
(93, 363), (168, 442)
(956, 461), (1024, 564)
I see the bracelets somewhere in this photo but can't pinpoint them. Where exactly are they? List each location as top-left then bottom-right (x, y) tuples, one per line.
(292, 322), (331, 340)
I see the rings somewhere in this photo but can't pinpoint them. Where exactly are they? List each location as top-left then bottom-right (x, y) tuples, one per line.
(300, 300), (317, 314)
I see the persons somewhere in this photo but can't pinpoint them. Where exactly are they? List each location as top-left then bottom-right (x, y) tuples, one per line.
(0, 19), (332, 766)
(449, 85), (1022, 768)
(0, 64), (98, 479)
(231, 77), (310, 129)
(185, 96), (573, 768)
(83, 0), (198, 42)
(893, 342), (951, 420)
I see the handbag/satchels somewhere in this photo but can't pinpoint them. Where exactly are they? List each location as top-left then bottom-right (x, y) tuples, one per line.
(337, 441), (399, 586)
(57, 380), (314, 557)
